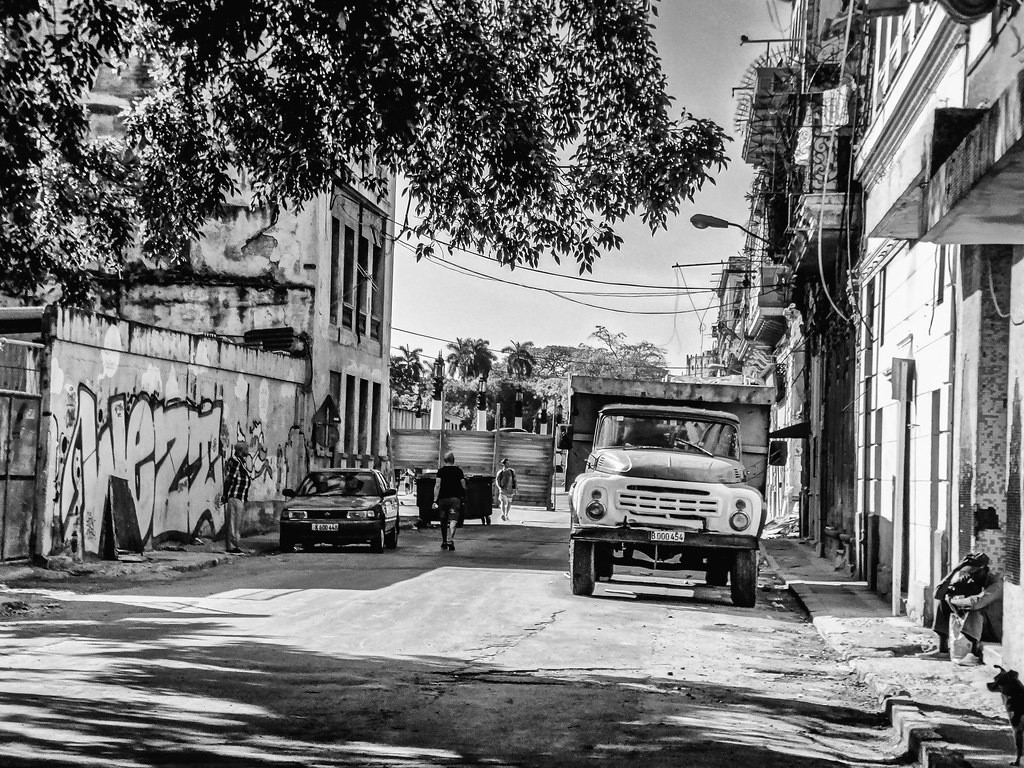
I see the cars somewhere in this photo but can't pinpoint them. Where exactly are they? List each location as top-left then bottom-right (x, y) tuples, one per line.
(276, 467), (400, 555)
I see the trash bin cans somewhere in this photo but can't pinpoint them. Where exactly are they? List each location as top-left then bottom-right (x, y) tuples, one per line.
(414, 474), (496, 525)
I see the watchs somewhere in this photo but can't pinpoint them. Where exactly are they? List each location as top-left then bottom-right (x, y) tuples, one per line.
(433, 500), (437, 504)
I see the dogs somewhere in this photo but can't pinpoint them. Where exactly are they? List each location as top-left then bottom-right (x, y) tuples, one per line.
(987, 664), (1024, 767)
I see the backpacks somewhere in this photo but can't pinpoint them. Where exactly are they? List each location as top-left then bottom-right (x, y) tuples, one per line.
(945, 552), (990, 618)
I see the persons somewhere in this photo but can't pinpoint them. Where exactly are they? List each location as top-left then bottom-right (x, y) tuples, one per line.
(915, 552), (1003, 665)
(432, 452), (467, 551)
(496, 459), (518, 522)
(221, 440), (251, 553)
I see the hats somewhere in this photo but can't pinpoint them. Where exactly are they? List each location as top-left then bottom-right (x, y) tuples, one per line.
(444, 452), (454, 459)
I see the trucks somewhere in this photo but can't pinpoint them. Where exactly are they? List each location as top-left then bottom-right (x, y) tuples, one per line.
(556, 374), (788, 608)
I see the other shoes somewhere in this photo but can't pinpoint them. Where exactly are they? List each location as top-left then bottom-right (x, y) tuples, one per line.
(447, 540), (455, 551)
(501, 515), (509, 521)
(225, 548), (245, 556)
(958, 652), (983, 666)
(441, 542), (447, 550)
(915, 649), (950, 661)
